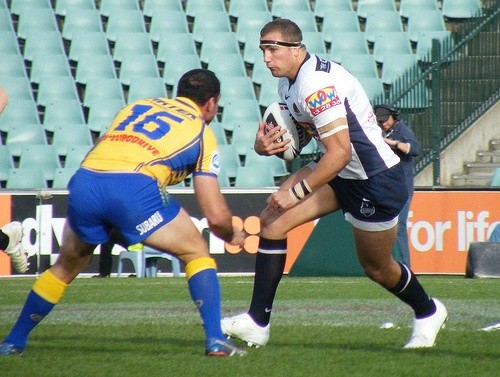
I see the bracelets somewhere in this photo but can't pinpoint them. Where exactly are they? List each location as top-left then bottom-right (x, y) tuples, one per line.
(288, 178), (313, 201)
(395, 140), (400, 147)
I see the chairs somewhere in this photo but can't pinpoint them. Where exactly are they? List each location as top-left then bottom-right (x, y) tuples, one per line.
(0, 0), (487, 191)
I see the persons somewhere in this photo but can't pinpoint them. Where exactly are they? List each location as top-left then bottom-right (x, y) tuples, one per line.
(218, 19), (448, 349)
(372, 104), (423, 271)
(0, 85), (28, 274)
(0, 68), (247, 358)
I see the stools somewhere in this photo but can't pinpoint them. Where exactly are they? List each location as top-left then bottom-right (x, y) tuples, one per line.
(118, 250), (180, 278)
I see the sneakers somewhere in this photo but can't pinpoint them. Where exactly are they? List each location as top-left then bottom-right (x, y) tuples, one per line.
(404, 297), (448, 349)
(205, 337), (247, 357)
(0, 341), (18, 356)
(221, 312), (271, 350)
(0, 221), (29, 274)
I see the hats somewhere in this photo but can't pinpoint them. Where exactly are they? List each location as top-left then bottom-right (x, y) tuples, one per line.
(376, 106), (390, 121)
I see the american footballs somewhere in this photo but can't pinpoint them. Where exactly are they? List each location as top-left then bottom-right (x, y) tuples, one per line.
(262, 101), (305, 161)
